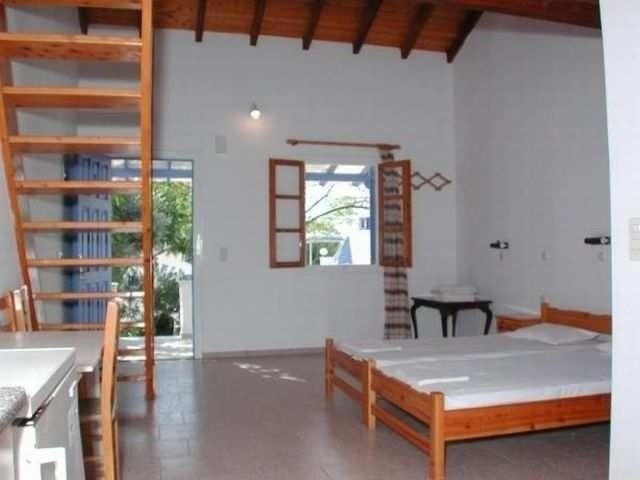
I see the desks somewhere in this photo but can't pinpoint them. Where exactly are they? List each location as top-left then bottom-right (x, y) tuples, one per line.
(0, 327), (105, 456)
(410, 294), (493, 337)
(0, 386), (27, 480)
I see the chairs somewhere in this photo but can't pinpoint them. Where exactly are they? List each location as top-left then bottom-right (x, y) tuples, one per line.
(8, 285), (34, 331)
(79, 298), (121, 480)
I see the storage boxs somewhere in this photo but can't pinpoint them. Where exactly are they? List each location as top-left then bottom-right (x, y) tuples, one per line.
(430, 285), (477, 301)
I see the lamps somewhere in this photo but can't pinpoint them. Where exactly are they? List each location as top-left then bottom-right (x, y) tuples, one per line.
(248, 103), (262, 120)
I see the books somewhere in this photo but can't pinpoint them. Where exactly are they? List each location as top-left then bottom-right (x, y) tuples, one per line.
(431, 295), (476, 303)
(430, 285), (477, 294)
